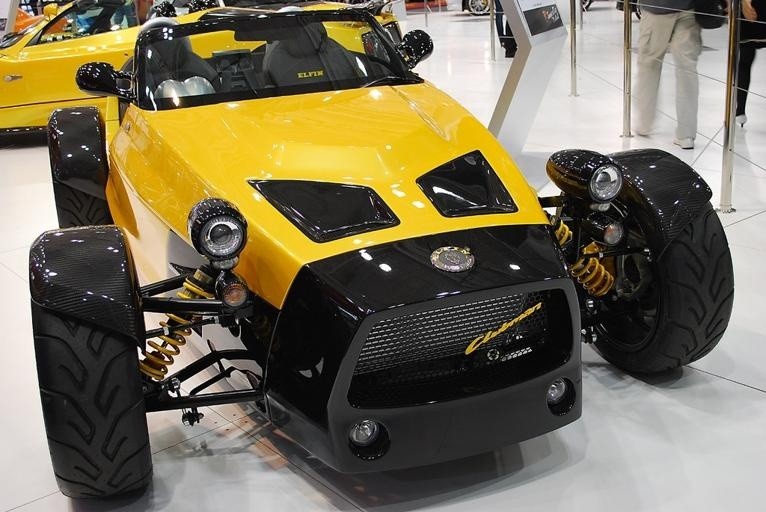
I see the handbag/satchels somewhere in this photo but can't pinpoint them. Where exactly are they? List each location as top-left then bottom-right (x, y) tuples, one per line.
(692, 0), (728, 29)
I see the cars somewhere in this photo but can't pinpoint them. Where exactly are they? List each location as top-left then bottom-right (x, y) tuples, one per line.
(0, 0), (409, 139)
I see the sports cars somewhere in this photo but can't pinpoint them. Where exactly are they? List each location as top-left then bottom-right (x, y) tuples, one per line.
(27, 0), (735, 502)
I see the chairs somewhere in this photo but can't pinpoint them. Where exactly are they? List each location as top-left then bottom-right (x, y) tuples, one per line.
(119, 6), (369, 117)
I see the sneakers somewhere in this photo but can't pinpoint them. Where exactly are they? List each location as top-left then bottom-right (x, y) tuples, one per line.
(673, 137), (694, 150)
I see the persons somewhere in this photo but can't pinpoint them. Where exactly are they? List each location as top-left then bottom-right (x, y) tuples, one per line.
(734, 0), (765, 129)
(631, 0), (700, 148)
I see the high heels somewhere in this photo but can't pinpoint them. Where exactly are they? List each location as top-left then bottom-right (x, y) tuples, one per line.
(735, 114), (747, 128)
